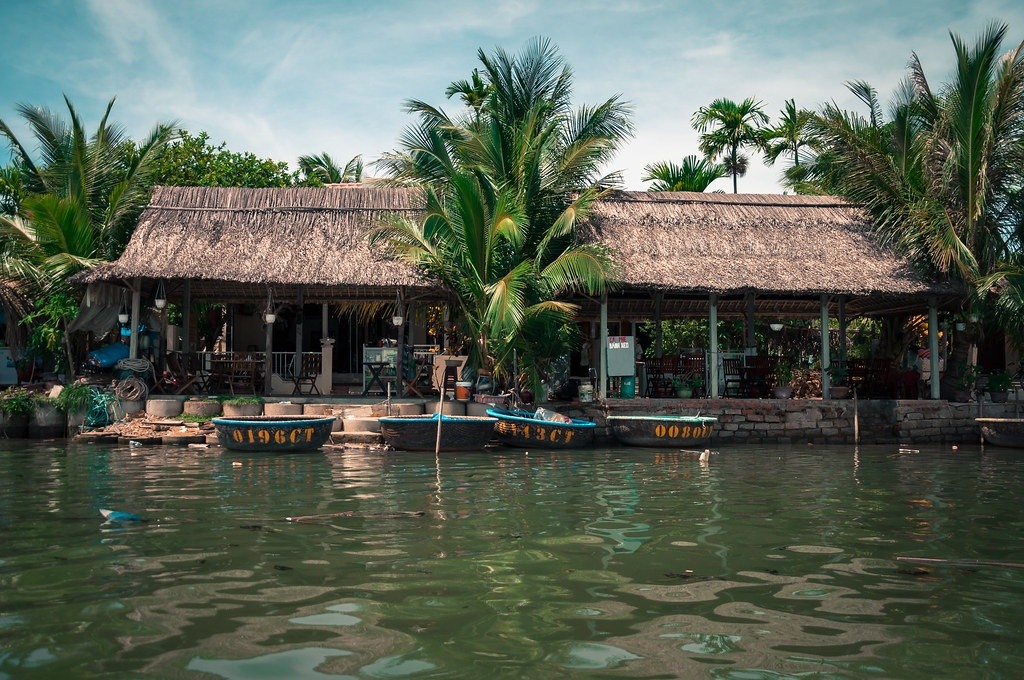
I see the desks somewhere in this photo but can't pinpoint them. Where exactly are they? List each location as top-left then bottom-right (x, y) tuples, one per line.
(207, 360), (266, 394)
(744, 379), (775, 399)
(362, 362), (390, 395)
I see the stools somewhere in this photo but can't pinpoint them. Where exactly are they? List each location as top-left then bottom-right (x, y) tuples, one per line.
(443, 366), (457, 397)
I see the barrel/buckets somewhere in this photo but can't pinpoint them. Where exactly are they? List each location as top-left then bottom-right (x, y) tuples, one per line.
(455, 382), (471, 401)
(577, 385), (594, 402)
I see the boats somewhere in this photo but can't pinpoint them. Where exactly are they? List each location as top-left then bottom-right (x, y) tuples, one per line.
(606, 414), (718, 447)
(974, 417), (1024, 447)
(485, 409), (596, 449)
(211, 413), (337, 453)
(377, 413), (497, 451)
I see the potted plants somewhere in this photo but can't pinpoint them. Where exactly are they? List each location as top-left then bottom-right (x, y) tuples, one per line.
(773, 362), (795, 399)
(825, 364), (849, 398)
(988, 368), (1013, 402)
(671, 378), (702, 399)
(1, 387), (502, 432)
(950, 360), (982, 402)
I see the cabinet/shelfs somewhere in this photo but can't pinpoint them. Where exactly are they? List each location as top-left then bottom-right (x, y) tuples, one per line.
(362, 344), (441, 395)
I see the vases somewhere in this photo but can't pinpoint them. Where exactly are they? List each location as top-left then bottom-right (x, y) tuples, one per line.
(155, 299), (167, 309)
(393, 317), (403, 325)
(266, 314), (276, 323)
(118, 314), (129, 323)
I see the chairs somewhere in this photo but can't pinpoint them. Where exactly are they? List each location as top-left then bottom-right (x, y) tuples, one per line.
(898, 370), (919, 399)
(721, 357), (746, 398)
(291, 355), (322, 397)
(140, 348), (267, 397)
(644, 350), (706, 398)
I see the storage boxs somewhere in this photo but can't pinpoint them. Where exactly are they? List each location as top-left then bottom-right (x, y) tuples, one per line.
(915, 356), (945, 385)
